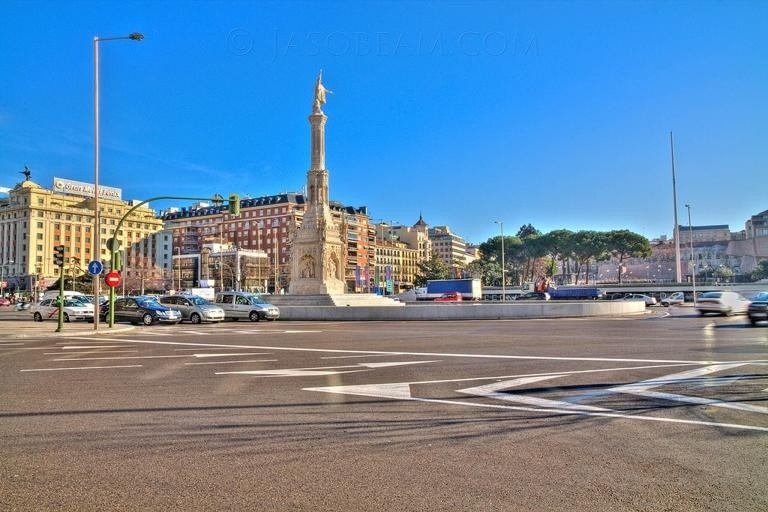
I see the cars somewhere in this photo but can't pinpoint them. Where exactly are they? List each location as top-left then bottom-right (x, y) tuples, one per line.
(99, 296), (182, 325)
(615, 293), (656, 307)
(0, 288), (161, 306)
(29, 298), (93, 323)
(433, 292), (462, 302)
(746, 291), (768, 327)
(695, 291), (750, 316)
(659, 292), (684, 307)
(515, 291), (551, 300)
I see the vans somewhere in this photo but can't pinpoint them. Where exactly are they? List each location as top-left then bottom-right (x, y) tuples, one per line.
(157, 294), (225, 323)
(213, 291), (280, 321)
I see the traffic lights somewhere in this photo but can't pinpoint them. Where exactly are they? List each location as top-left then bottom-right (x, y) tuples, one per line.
(52, 245), (65, 267)
(215, 260), (218, 270)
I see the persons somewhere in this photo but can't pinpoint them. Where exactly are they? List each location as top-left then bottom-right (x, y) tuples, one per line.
(312, 69), (334, 115)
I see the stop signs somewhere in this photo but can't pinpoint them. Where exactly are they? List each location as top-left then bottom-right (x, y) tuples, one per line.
(106, 273), (121, 286)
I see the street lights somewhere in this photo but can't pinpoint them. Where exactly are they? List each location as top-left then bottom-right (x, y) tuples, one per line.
(683, 202), (695, 304)
(0, 260), (14, 295)
(251, 222), (261, 292)
(173, 246), (181, 292)
(368, 218), (385, 297)
(94, 32), (145, 330)
(492, 219), (506, 302)
(389, 219), (399, 295)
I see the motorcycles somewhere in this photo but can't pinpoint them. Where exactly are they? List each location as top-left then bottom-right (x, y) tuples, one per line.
(12, 302), (30, 311)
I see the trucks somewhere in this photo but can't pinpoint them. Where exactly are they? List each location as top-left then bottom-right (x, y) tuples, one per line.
(416, 278), (482, 302)
(534, 274), (602, 300)
